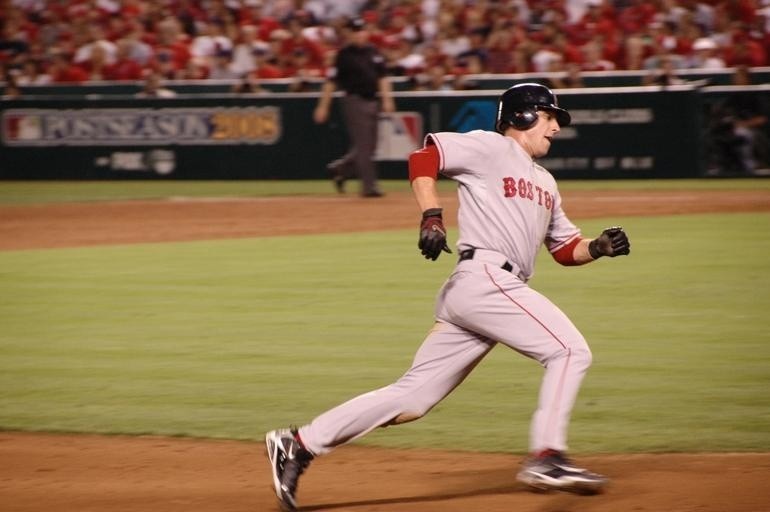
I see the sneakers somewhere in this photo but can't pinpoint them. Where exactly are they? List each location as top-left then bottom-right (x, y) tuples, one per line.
(327, 163), (386, 199)
(513, 448), (607, 495)
(264, 425), (313, 512)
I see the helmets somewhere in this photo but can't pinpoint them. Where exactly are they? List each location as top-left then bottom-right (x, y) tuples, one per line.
(495, 80), (574, 133)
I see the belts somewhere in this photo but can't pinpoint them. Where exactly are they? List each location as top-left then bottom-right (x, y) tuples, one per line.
(457, 249), (512, 273)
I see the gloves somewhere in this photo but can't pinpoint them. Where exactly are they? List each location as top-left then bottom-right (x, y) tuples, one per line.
(588, 224), (630, 260)
(416, 205), (453, 262)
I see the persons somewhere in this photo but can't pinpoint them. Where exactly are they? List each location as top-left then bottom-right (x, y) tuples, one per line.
(314, 16), (398, 197)
(0, 0), (770, 110)
(266, 81), (634, 511)
